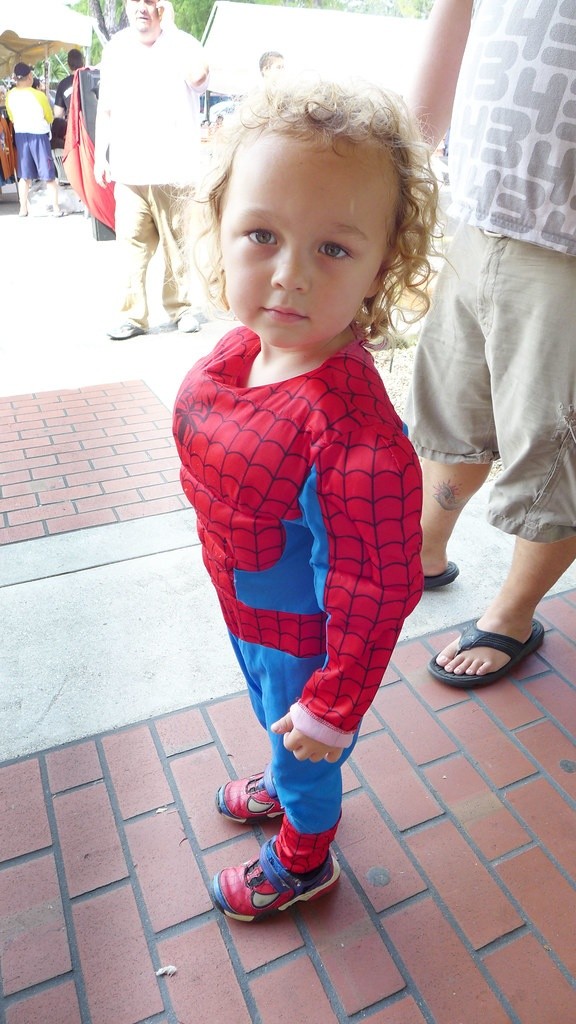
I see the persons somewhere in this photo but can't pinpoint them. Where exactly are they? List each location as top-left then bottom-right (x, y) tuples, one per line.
(5, 63), (69, 217)
(92, 0), (208, 335)
(32, 78), (54, 109)
(170, 81), (440, 922)
(260, 52), (284, 76)
(54, 49), (84, 120)
(410, 0), (576, 685)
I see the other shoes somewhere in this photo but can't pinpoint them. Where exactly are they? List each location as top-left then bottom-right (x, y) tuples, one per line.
(53, 210), (65, 217)
(18, 211), (28, 217)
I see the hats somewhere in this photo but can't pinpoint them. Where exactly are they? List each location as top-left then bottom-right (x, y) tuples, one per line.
(14, 62), (35, 78)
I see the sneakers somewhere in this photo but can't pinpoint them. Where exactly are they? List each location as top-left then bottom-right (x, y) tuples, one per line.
(210, 834), (341, 922)
(214, 764), (286, 825)
(106, 321), (146, 339)
(177, 314), (200, 335)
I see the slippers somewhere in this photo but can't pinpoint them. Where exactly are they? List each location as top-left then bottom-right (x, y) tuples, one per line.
(423, 560), (460, 589)
(427, 614), (545, 687)
